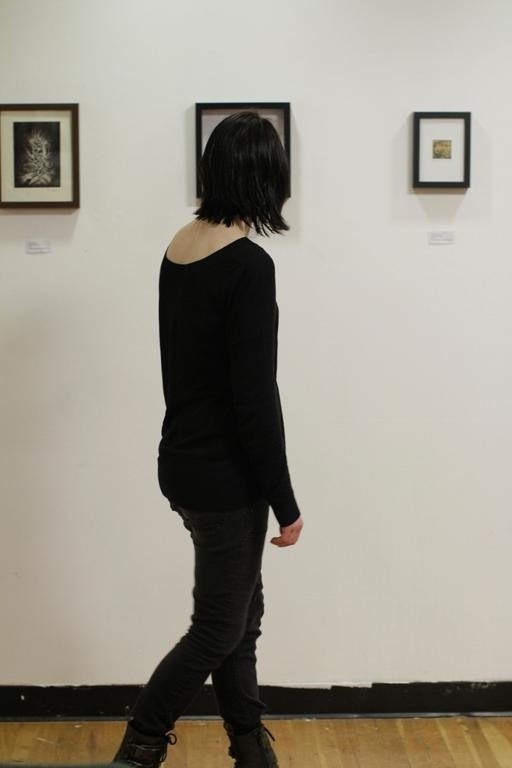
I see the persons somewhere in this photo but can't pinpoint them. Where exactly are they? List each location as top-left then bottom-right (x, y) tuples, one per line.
(102, 112), (306, 767)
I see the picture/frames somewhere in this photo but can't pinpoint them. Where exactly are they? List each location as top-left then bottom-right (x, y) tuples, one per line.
(413, 111), (471, 189)
(195, 101), (291, 199)
(0, 102), (79, 208)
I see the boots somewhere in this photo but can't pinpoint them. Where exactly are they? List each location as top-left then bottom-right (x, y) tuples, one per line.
(110, 722), (177, 768)
(223, 721), (279, 768)
